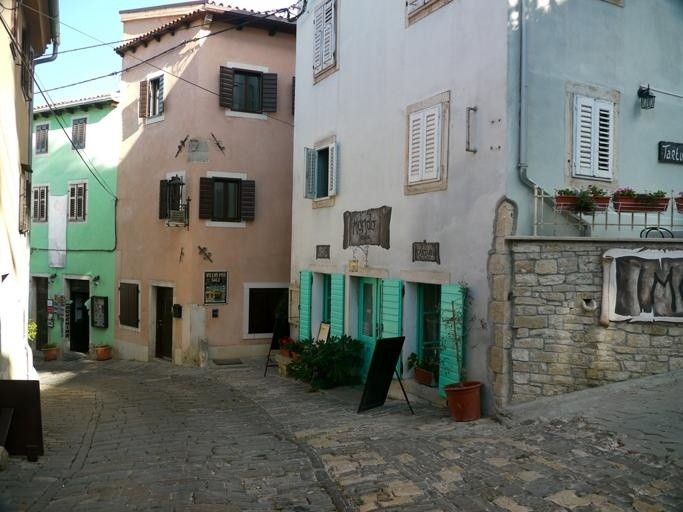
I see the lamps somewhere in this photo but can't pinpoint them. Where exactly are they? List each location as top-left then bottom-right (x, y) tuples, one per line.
(346, 241), (368, 273)
(636, 84), (655, 111)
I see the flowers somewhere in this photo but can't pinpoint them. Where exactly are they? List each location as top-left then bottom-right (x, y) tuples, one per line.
(611, 186), (635, 200)
(277, 336), (294, 350)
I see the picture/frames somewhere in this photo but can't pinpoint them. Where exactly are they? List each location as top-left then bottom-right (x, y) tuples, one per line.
(203, 270), (226, 303)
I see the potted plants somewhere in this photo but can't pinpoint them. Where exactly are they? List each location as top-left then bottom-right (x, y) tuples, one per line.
(406, 353), (438, 387)
(90, 342), (111, 360)
(40, 342), (56, 362)
(438, 279), (487, 422)
(554, 185), (611, 212)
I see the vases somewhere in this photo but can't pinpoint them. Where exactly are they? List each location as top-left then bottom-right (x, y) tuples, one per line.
(90, 295), (108, 328)
(279, 347), (291, 357)
(614, 196), (670, 212)
